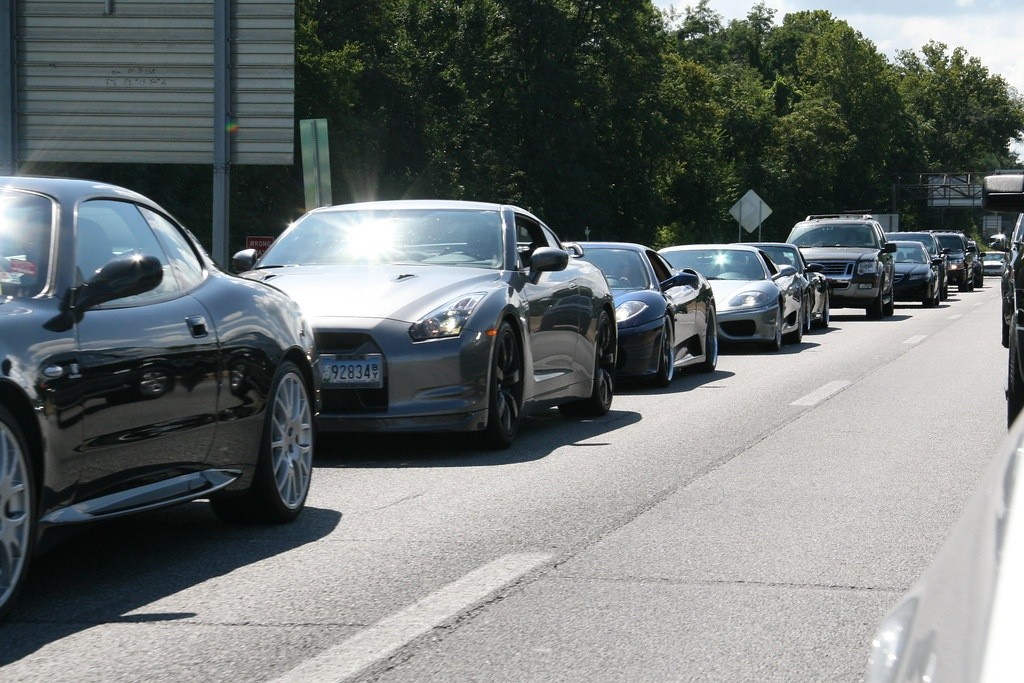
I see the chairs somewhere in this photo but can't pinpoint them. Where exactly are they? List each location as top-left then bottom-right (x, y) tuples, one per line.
(23, 216), (111, 296)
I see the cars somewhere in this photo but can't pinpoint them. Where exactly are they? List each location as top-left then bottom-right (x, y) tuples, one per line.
(968, 241), (986, 288)
(561, 242), (718, 388)
(0, 177), (322, 627)
(657, 244), (805, 352)
(885, 241), (940, 308)
(980, 251), (1008, 275)
(735, 242), (830, 333)
(230, 200), (618, 450)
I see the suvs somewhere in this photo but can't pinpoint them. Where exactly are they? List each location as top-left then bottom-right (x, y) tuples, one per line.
(884, 230), (948, 301)
(786, 214), (897, 321)
(930, 229), (974, 292)
